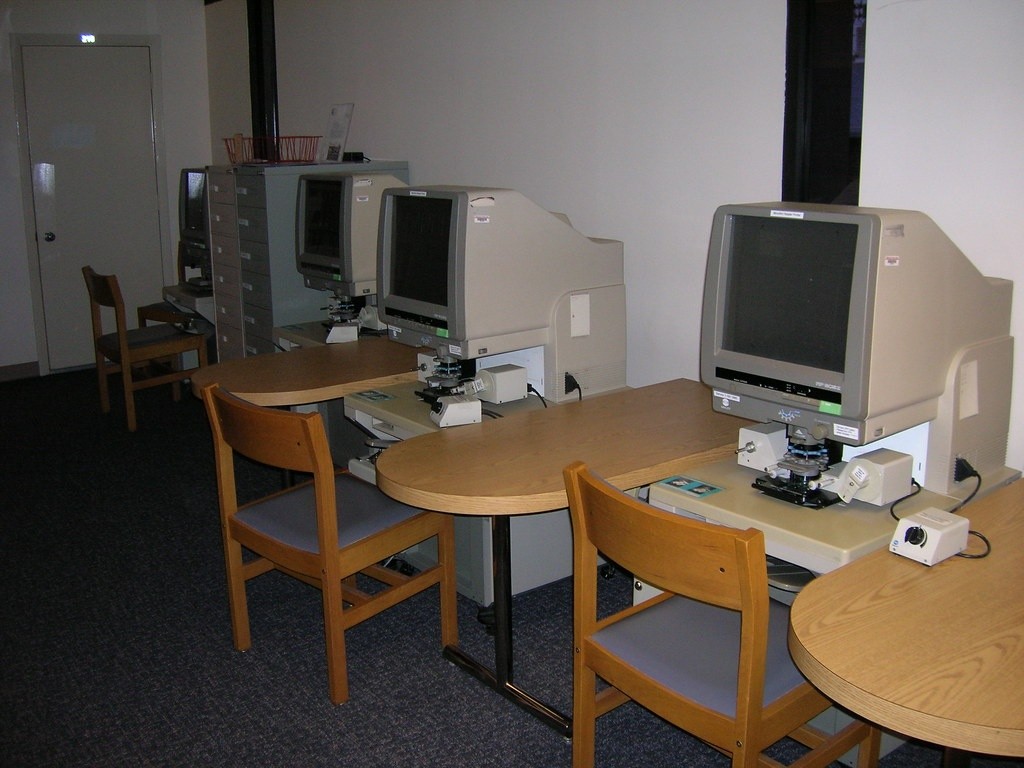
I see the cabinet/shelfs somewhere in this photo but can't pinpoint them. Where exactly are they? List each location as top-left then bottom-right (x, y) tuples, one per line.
(205, 159), (410, 364)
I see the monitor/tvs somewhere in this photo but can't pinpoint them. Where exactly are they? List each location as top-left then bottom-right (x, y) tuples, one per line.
(375, 184), (624, 361)
(701, 201), (1013, 448)
(178, 169), (207, 241)
(295, 169), (410, 298)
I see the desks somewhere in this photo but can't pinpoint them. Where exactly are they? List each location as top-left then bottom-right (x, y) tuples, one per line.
(137, 301), (206, 374)
(633, 456), (1024, 768)
(189, 337), (429, 490)
(273, 321), (386, 471)
(787, 476), (1024, 768)
(375, 378), (758, 745)
(161, 285), (215, 384)
(344, 380), (637, 608)
(343, 385), (635, 605)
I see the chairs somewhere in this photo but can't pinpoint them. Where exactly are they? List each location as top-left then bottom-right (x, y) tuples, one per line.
(81, 264), (208, 433)
(563, 461), (883, 768)
(198, 382), (457, 707)
